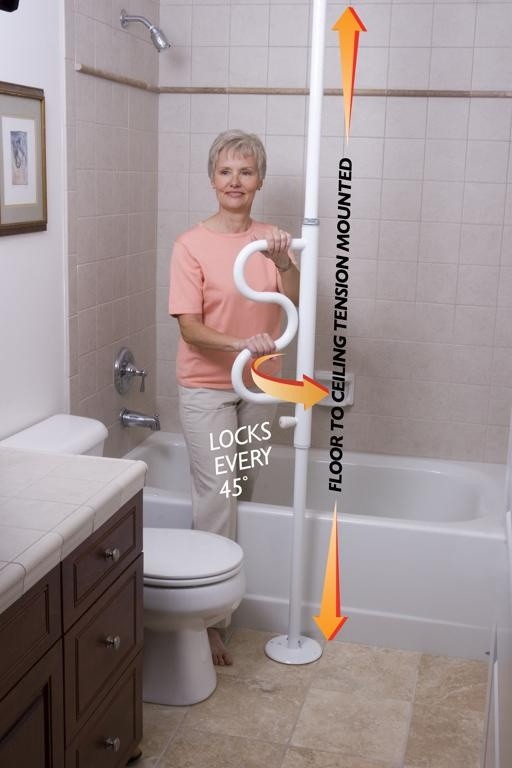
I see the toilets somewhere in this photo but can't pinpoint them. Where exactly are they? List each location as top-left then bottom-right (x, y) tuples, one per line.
(0, 411), (247, 708)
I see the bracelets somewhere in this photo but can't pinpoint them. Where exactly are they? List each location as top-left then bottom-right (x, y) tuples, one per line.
(275, 257), (292, 272)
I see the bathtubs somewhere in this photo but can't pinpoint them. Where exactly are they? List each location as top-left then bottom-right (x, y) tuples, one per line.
(118, 431), (512, 663)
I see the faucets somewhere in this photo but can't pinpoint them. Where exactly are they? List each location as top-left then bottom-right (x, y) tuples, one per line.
(119, 408), (161, 432)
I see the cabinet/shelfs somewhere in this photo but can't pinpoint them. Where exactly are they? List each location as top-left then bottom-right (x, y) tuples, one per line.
(1, 492), (145, 767)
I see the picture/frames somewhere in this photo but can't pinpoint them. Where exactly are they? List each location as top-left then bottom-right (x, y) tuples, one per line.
(0, 80), (46, 235)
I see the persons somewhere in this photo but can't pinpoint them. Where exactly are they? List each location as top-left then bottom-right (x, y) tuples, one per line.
(165, 126), (301, 665)
